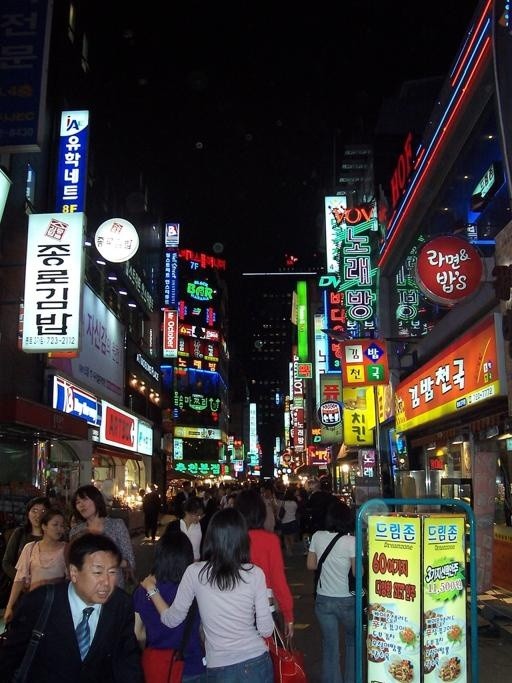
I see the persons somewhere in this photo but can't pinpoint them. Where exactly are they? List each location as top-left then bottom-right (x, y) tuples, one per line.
(0, 476), (367, 680)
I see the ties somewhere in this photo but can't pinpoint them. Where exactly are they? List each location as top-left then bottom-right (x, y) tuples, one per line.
(76, 607), (94, 663)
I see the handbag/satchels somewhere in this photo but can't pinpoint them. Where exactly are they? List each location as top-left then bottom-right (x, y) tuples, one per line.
(140, 648), (185, 683)
(279, 506), (285, 519)
(262, 627), (309, 683)
